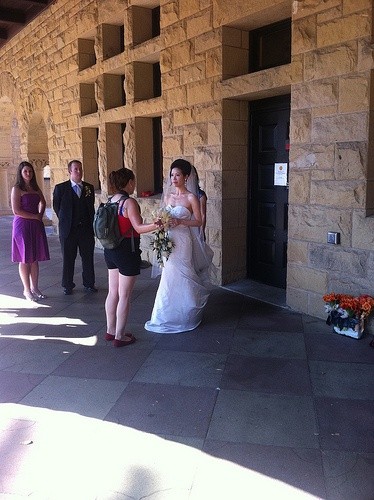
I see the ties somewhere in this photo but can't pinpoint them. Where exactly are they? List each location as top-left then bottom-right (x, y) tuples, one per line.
(76, 184), (82, 199)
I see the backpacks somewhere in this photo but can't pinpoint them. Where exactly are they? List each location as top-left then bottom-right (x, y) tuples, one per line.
(92, 194), (141, 250)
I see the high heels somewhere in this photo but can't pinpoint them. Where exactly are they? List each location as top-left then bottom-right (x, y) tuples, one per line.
(31, 289), (46, 299)
(23, 293), (38, 302)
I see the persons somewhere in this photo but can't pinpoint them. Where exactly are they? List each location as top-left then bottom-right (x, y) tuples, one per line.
(11, 161), (51, 302)
(104, 168), (164, 347)
(53, 160), (97, 294)
(192, 165), (207, 241)
(144, 158), (218, 334)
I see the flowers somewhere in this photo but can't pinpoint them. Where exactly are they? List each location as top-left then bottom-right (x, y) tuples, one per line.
(322, 291), (374, 331)
(149, 209), (176, 268)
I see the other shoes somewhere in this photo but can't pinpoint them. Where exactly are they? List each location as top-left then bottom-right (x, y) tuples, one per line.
(105, 332), (133, 341)
(86, 287), (97, 293)
(63, 288), (73, 296)
(113, 336), (136, 347)
